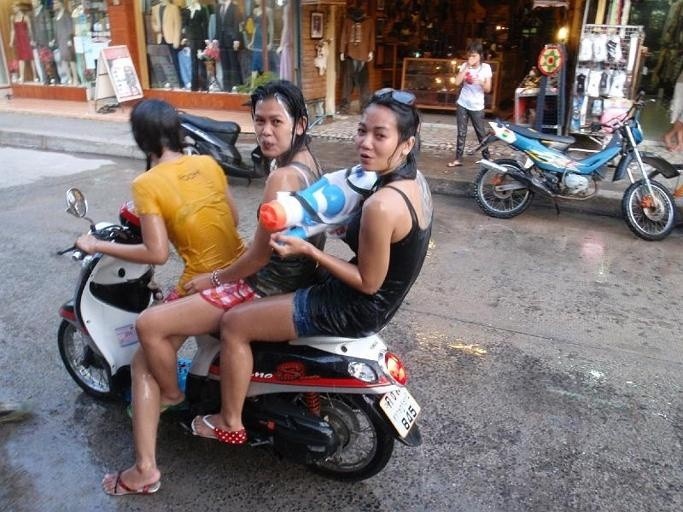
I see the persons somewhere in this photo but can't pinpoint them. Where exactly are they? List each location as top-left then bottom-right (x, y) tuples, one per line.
(74, 96), (251, 495)
(127, 79), (324, 420)
(5, 0), (294, 91)
(186, 85), (436, 447)
(659, 69), (682, 154)
(446, 42), (493, 166)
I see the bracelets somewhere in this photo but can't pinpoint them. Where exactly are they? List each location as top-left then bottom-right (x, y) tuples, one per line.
(213, 269), (222, 286)
(93, 239), (101, 252)
(210, 272), (217, 289)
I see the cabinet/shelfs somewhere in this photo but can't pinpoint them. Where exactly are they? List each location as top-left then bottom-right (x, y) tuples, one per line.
(399, 56), (501, 116)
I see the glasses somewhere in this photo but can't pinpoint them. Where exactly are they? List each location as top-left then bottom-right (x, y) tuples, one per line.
(373, 88), (417, 106)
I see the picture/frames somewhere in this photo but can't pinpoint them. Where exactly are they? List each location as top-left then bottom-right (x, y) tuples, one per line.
(309, 11), (324, 39)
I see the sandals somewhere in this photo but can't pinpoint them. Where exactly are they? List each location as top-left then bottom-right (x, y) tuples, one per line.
(191, 414), (247, 447)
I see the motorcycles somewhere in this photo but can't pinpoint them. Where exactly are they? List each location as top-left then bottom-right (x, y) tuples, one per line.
(176, 100), (271, 184)
(470, 87), (681, 241)
(52, 185), (426, 484)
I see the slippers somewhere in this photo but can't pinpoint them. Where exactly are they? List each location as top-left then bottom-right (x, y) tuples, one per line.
(447, 161), (463, 167)
(126, 393), (188, 419)
(102, 470), (161, 496)
